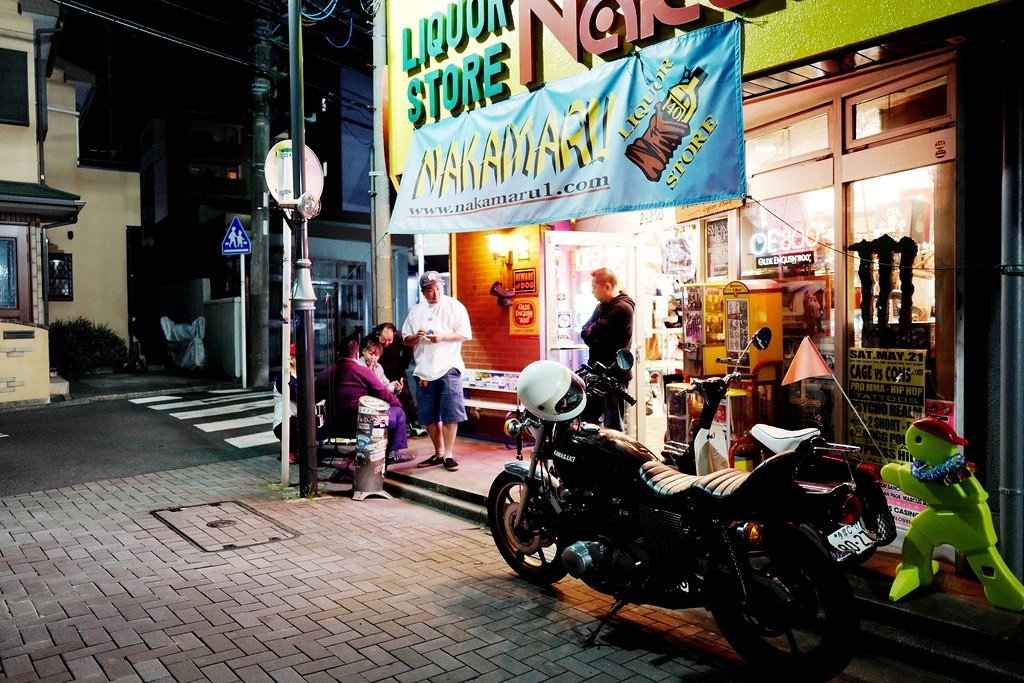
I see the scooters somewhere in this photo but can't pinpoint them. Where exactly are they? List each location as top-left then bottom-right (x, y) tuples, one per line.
(659, 327), (897, 568)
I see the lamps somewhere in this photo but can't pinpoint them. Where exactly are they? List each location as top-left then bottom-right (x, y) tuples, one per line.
(487, 233), (512, 268)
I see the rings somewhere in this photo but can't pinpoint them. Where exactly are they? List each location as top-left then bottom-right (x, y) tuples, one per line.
(398, 387), (399, 388)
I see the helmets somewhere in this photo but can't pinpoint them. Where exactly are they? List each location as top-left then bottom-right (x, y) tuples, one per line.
(517, 360), (588, 421)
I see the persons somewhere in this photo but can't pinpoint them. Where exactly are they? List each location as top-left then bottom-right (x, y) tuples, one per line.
(315, 338), (416, 464)
(357, 338), (404, 399)
(401, 271), (472, 471)
(580, 268), (636, 432)
(359, 323), (428, 438)
(272, 342), (300, 463)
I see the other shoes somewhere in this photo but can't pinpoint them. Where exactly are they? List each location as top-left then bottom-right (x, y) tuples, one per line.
(390, 451), (415, 462)
(443, 458), (459, 470)
(289, 453), (296, 463)
(417, 455), (444, 467)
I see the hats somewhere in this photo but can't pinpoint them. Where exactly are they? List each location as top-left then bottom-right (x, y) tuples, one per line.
(420, 270), (441, 288)
(290, 344), (296, 355)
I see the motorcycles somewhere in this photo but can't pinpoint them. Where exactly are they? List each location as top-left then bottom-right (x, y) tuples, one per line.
(486, 349), (877, 683)
